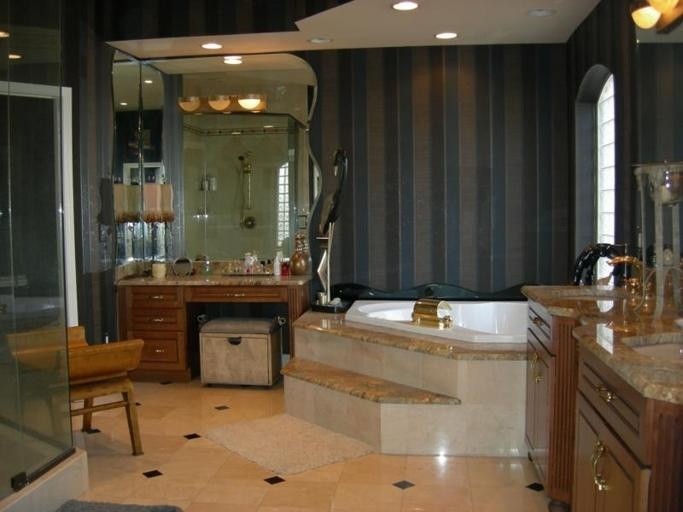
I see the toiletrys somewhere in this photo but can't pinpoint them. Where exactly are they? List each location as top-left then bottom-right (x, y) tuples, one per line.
(227, 252), (290, 276)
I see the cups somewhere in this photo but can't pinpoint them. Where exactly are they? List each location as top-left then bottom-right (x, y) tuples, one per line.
(152, 264), (166, 279)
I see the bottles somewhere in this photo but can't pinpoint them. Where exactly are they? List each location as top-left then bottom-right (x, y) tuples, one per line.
(273, 240), (308, 276)
(244, 253), (251, 274)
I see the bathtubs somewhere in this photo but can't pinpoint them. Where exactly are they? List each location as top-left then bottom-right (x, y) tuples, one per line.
(345, 296), (532, 352)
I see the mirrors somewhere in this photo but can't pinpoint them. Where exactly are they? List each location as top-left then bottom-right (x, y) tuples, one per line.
(110, 47), (166, 261)
(181, 111), (298, 261)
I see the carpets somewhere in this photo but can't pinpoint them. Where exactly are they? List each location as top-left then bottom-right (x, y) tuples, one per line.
(208, 412), (374, 479)
(58, 499), (183, 512)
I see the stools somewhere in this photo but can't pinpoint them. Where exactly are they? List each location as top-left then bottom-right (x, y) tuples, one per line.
(8, 325), (145, 459)
(199, 317), (282, 388)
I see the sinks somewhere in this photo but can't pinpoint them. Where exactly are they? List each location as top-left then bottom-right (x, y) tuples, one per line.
(551, 286), (635, 303)
(221, 272), (274, 278)
(620, 326), (682, 364)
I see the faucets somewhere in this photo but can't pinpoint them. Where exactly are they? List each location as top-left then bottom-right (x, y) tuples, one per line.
(196, 260), (211, 273)
(423, 282), (442, 298)
(605, 255), (653, 295)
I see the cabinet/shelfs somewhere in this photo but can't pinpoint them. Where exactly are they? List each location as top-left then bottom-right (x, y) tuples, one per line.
(524, 297), (682, 512)
(118, 284), (310, 384)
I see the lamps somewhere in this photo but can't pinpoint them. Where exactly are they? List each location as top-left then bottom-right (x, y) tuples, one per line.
(143, 173), (174, 224)
(179, 93), (265, 112)
(114, 176), (141, 224)
